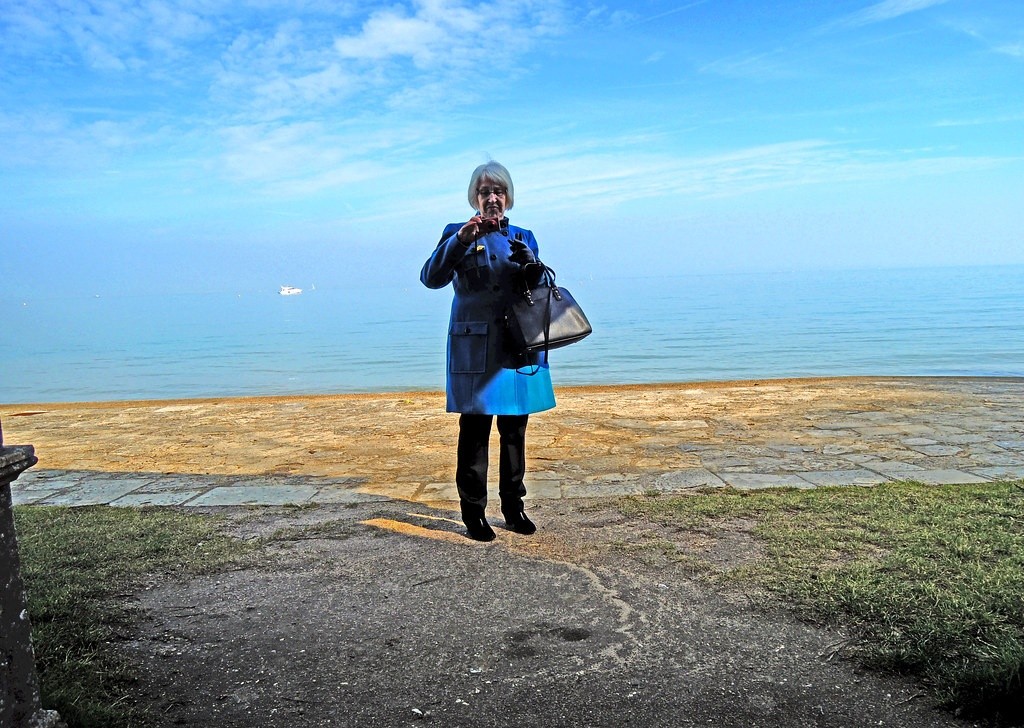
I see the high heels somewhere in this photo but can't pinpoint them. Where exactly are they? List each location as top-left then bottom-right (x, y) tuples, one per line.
(501, 502), (536, 534)
(461, 508), (495, 541)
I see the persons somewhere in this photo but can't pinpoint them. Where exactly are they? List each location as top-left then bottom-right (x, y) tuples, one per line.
(418, 161), (556, 543)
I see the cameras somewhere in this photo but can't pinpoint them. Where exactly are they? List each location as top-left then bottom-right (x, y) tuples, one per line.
(476, 216), (501, 234)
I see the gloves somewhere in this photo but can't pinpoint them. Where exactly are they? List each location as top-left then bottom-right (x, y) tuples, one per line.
(507, 233), (536, 266)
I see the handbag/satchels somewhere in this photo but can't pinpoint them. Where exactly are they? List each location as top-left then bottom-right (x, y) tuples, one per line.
(505, 256), (592, 357)
